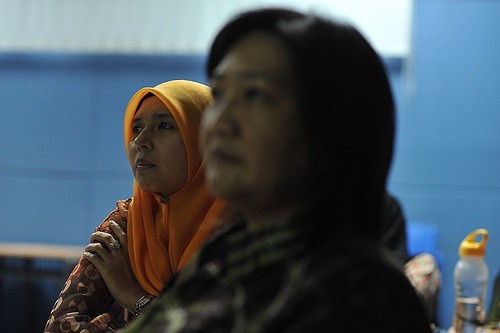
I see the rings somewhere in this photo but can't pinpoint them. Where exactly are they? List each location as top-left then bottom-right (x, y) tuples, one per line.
(108, 240), (121, 251)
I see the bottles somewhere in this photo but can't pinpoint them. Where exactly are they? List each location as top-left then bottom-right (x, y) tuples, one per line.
(453, 228), (489, 333)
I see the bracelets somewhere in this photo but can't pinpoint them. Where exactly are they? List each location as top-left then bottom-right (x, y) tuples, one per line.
(132, 293), (155, 317)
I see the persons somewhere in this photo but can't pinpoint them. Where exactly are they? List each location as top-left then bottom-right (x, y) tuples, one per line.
(116, 9), (432, 333)
(44, 80), (227, 333)
